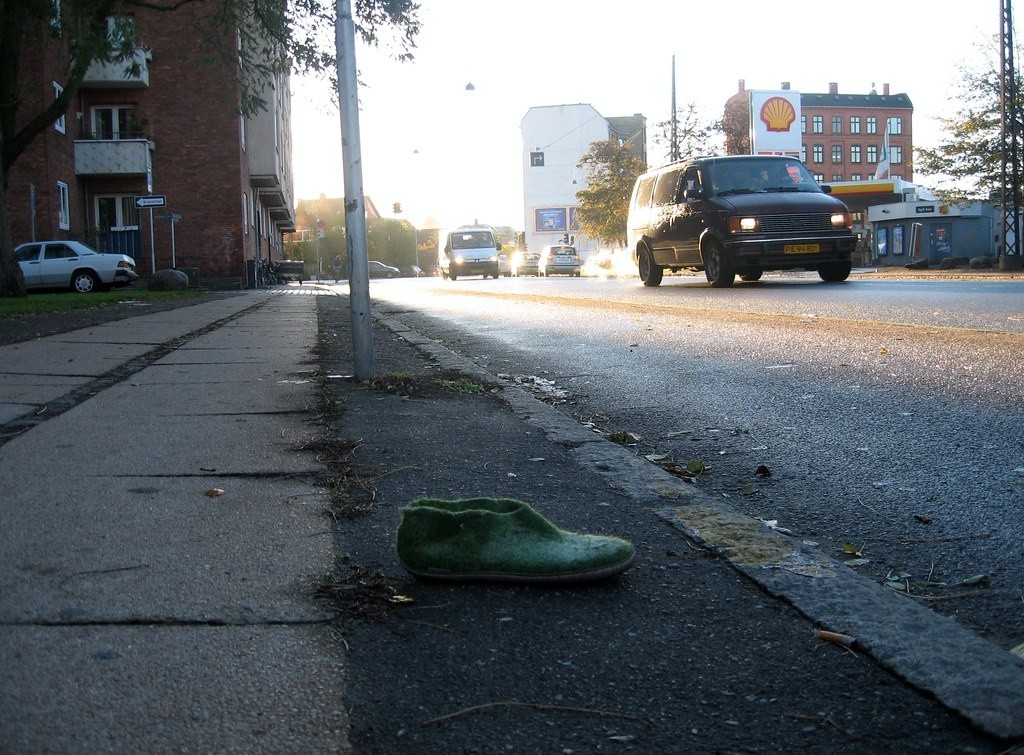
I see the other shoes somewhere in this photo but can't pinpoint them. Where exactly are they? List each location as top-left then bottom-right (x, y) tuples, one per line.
(396, 497), (637, 585)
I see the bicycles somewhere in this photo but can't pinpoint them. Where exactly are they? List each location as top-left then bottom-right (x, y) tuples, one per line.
(252, 255), (287, 284)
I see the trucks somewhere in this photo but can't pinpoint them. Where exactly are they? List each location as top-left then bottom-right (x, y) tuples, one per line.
(439, 228), (502, 280)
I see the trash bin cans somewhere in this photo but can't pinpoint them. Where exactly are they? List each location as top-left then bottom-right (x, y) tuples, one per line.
(176, 266), (201, 288)
(278, 260), (304, 285)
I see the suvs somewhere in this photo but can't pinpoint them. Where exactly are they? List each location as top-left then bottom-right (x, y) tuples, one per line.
(626, 154), (859, 287)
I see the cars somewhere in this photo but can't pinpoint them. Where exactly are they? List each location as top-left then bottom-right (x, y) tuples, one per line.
(368, 260), (400, 278)
(13, 239), (140, 293)
(500, 251), (542, 277)
(537, 245), (581, 277)
(400, 264), (440, 277)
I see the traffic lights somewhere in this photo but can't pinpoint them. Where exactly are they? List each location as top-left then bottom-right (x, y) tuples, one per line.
(513, 231), (527, 251)
(393, 203), (401, 214)
(564, 234), (569, 243)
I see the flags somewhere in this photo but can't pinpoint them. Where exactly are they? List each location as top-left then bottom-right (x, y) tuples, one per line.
(873, 124), (889, 180)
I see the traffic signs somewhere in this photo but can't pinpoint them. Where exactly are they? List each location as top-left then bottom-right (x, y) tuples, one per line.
(134, 195), (166, 209)
